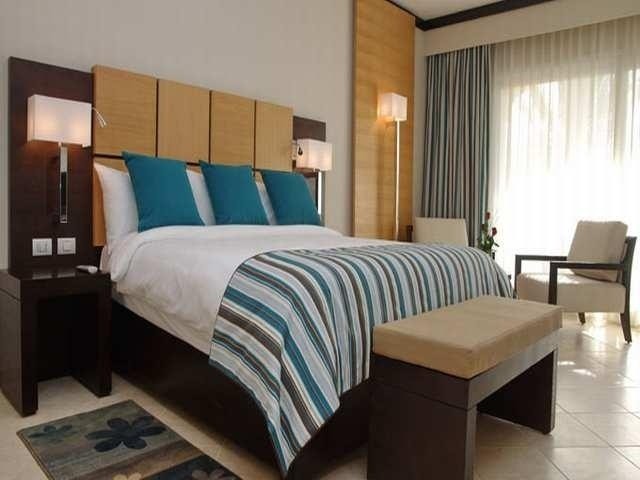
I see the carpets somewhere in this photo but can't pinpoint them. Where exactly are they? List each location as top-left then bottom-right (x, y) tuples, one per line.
(16, 399), (242, 480)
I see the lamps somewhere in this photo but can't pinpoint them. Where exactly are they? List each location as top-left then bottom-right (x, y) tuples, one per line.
(26, 94), (91, 148)
(377, 93), (408, 122)
(296, 139), (333, 172)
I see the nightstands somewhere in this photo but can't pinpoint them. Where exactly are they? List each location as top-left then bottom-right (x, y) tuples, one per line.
(1, 268), (111, 417)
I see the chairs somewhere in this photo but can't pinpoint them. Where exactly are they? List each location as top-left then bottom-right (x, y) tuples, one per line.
(513, 220), (637, 344)
(406, 215), (469, 250)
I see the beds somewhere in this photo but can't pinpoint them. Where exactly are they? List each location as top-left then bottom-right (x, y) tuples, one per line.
(7, 55), (513, 480)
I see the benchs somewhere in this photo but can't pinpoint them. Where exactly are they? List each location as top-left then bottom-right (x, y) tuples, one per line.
(366, 294), (564, 480)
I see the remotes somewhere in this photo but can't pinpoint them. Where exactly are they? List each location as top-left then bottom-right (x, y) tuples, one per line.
(76, 264), (98, 274)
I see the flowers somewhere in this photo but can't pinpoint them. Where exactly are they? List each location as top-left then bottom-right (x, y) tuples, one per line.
(477, 213), (499, 255)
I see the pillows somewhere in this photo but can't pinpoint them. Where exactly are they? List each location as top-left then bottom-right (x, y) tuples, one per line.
(259, 167), (320, 225)
(94, 161), (139, 255)
(122, 150), (205, 233)
(199, 158), (268, 224)
